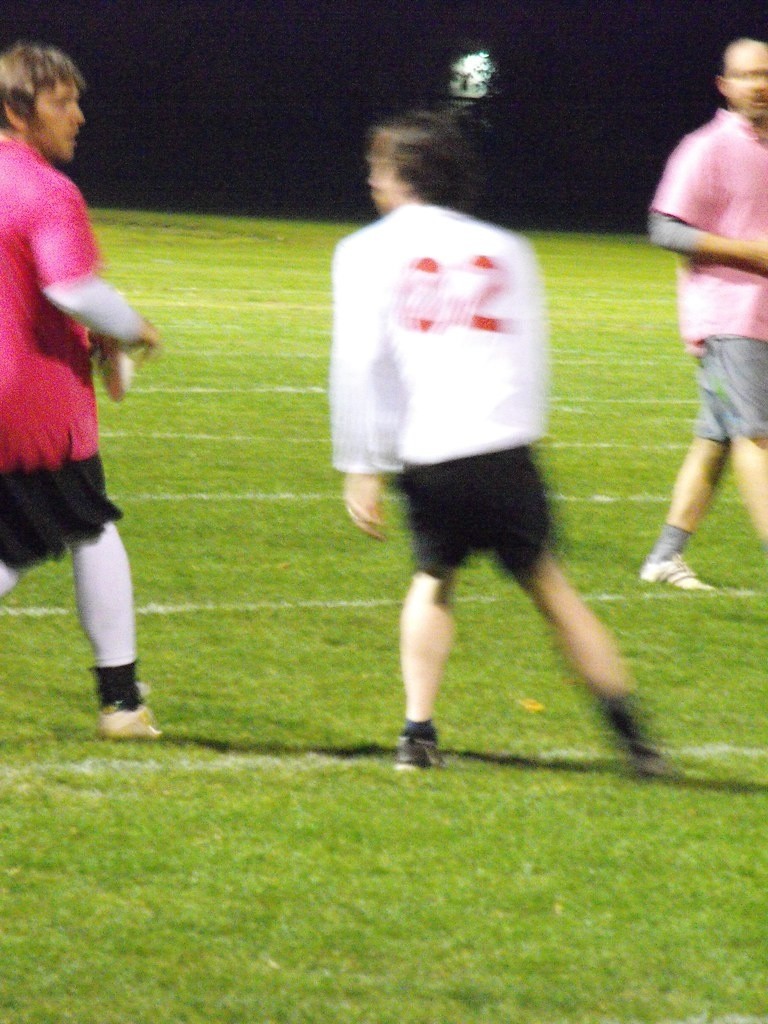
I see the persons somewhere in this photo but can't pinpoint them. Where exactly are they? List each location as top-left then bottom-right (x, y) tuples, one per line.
(331, 114), (679, 783)
(0, 43), (163, 741)
(639, 33), (768, 595)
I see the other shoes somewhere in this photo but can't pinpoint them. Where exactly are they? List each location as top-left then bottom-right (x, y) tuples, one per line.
(637, 758), (678, 782)
(97, 680), (163, 740)
(396, 726), (448, 771)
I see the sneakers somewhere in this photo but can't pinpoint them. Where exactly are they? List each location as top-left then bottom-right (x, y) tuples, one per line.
(641, 553), (714, 590)
